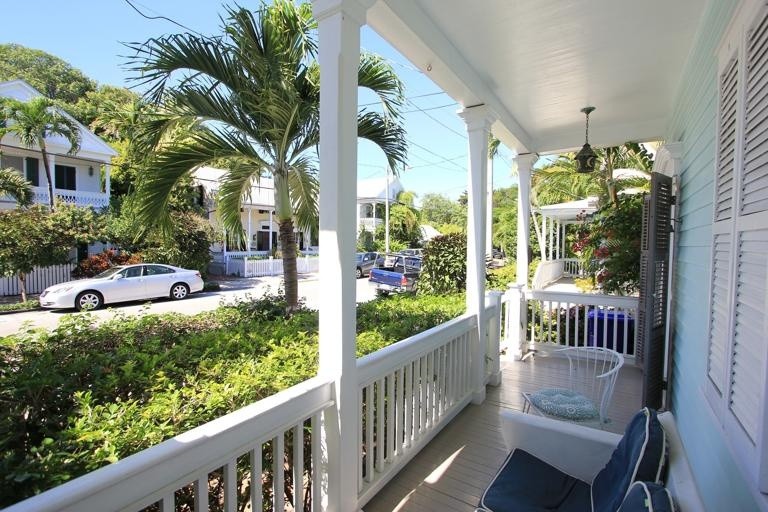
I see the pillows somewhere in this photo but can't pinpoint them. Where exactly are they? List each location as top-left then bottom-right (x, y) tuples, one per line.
(590, 407), (671, 512)
(616, 480), (675, 512)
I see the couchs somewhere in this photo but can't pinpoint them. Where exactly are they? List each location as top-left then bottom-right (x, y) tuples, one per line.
(474, 407), (708, 512)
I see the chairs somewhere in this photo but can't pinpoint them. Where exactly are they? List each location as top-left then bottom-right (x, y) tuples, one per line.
(520, 347), (624, 430)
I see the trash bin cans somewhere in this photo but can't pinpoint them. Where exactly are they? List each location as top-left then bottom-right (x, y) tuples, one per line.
(587, 309), (635, 354)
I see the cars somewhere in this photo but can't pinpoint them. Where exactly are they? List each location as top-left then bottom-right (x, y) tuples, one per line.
(399, 249), (427, 258)
(356, 252), (385, 279)
(39, 263), (204, 311)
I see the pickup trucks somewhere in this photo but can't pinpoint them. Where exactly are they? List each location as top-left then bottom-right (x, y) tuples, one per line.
(369, 256), (423, 299)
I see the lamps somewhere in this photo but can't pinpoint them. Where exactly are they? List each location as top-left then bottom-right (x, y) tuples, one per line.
(574, 107), (599, 173)
